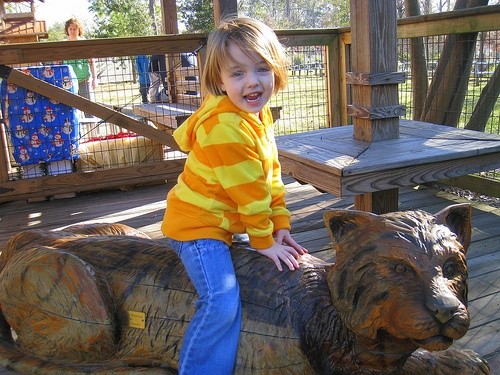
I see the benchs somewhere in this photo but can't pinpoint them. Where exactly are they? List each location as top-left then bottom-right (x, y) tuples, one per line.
(133, 66), (215, 129)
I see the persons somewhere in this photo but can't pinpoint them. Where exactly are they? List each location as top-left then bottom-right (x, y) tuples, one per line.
(136, 54), (199, 103)
(62, 19), (97, 117)
(160, 16), (309, 375)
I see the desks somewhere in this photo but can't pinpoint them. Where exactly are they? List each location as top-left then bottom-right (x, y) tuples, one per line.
(266, 116), (500, 199)
